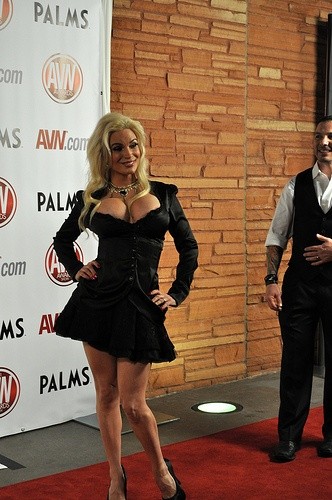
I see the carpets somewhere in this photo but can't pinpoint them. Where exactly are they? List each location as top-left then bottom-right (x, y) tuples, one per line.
(0, 406), (332, 500)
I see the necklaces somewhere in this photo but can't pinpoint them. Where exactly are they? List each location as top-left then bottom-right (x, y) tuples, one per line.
(108, 179), (141, 198)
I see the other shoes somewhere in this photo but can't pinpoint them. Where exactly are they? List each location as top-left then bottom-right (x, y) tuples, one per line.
(317, 437), (331, 457)
(274, 434), (301, 460)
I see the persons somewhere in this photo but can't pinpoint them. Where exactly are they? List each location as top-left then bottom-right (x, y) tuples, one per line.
(53, 112), (199, 500)
(264, 115), (332, 462)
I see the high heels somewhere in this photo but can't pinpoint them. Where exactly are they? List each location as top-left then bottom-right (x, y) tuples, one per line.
(106, 464), (127, 499)
(161, 457), (186, 499)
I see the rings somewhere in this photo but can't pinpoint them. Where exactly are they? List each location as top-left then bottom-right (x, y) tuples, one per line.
(162, 297), (166, 303)
(316, 256), (321, 261)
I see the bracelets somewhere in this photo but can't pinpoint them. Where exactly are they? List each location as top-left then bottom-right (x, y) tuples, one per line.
(263, 273), (278, 287)
